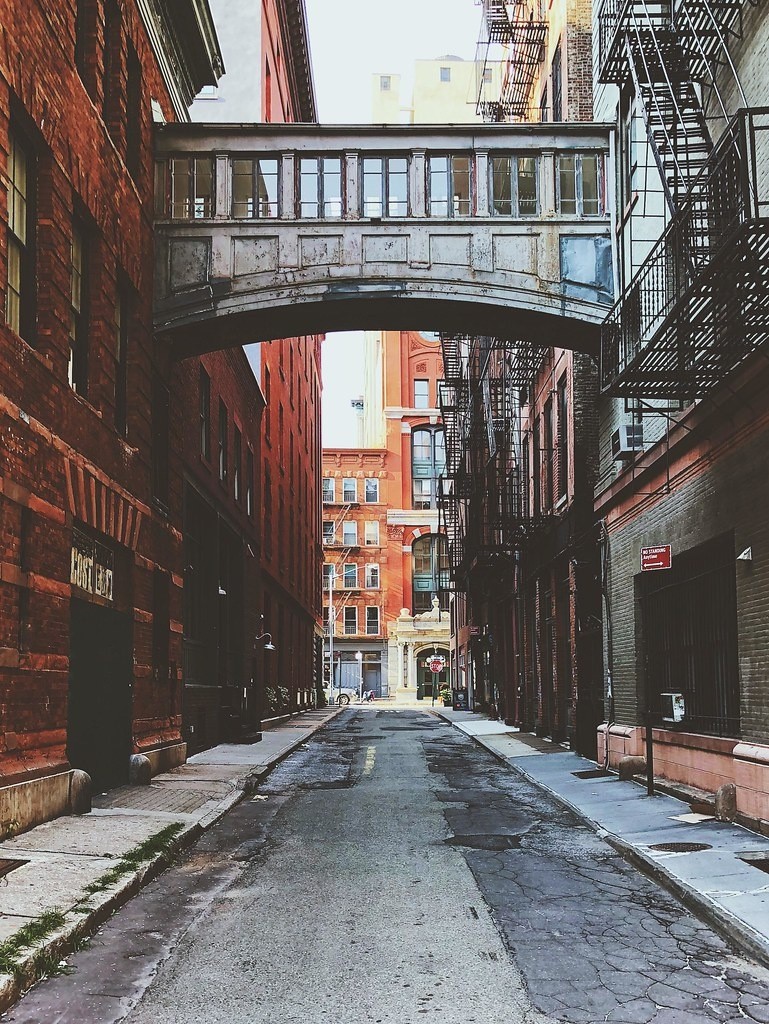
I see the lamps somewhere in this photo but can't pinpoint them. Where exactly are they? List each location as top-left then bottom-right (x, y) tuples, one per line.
(255, 633), (276, 651)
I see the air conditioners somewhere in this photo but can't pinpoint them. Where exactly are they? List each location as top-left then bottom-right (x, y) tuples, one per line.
(610, 424), (643, 461)
(324, 538), (333, 545)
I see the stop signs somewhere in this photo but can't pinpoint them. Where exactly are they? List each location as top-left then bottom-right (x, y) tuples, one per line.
(430, 660), (443, 673)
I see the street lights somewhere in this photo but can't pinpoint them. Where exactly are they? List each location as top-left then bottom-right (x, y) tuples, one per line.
(329, 564), (378, 705)
(426, 655), (445, 707)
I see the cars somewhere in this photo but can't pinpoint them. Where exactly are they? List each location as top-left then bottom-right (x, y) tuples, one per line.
(322, 681), (358, 705)
